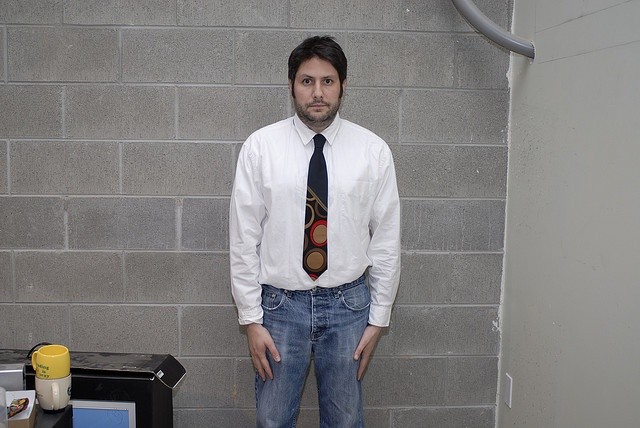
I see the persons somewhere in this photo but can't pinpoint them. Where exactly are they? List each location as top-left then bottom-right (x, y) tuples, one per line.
(229, 36), (401, 428)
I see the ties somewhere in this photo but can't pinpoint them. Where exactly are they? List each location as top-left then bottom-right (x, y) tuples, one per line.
(302, 134), (329, 283)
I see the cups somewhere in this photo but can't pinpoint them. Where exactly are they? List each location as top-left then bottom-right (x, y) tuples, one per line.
(31, 344), (71, 380)
(33, 373), (73, 411)
